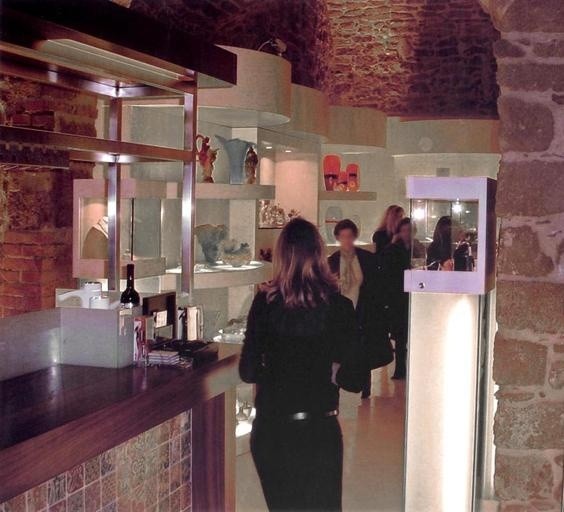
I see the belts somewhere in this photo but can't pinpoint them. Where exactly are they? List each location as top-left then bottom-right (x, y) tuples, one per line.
(257, 410), (339, 421)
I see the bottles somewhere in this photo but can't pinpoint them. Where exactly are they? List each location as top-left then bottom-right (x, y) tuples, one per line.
(466, 246), (474, 271)
(121, 263), (139, 309)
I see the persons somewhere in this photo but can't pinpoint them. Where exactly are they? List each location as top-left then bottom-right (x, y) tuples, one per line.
(327, 221), (383, 399)
(377, 219), (427, 380)
(240, 219), (370, 511)
(427, 217), (470, 272)
(374, 205), (406, 262)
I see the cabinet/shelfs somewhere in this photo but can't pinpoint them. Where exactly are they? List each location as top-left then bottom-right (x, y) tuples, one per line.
(0, 35), (243, 512)
(320, 107), (386, 255)
(112, 43), (294, 459)
(260, 87), (328, 265)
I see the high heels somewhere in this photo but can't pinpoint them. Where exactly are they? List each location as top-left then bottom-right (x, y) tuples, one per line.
(361, 383), (371, 398)
(391, 367), (405, 379)
